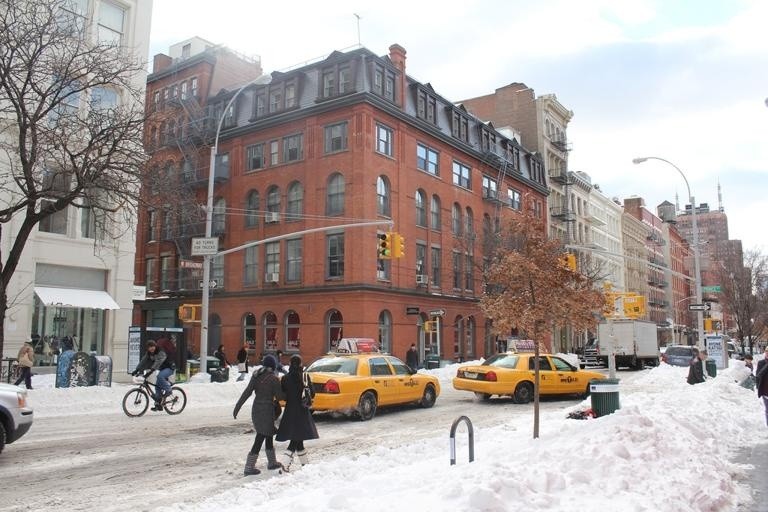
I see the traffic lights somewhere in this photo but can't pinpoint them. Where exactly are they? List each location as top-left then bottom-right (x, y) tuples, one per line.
(394, 234), (405, 258)
(378, 233), (390, 260)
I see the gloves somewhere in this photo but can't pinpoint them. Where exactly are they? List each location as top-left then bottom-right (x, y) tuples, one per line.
(142, 371), (153, 378)
(132, 370), (139, 376)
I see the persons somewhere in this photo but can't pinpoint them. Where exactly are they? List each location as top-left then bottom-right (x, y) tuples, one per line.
(14, 335), (34, 389)
(744, 354), (754, 372)
(274, 353), (320, 474)
(756, 345), (768, 429)
(70, 334), (80, 352)
(236, 344), (249, 381)
(275, 349), (289, 377)
(214, 345), (232, 369)
(405, 342), (420, 371)
(132, 339), (177, 411)
(686, 350), (709, 385)
(232, 353), (288, 477)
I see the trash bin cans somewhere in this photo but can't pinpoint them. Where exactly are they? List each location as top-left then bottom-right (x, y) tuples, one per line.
(589, 378), (620, 418)
(707, 362), (716, 377)
(706, 360), (715, 370)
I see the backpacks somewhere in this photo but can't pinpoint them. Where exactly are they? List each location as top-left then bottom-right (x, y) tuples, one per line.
(155, 339), (177, 370)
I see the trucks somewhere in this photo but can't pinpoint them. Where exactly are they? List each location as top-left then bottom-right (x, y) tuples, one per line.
(598, 319), (661, 370)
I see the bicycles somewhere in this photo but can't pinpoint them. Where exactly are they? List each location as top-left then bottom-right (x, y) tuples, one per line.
(120, 370), (187, 417)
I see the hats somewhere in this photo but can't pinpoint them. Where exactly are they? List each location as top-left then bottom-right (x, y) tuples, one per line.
(263, 355), (276, 371)
(25, 339), (32, 343)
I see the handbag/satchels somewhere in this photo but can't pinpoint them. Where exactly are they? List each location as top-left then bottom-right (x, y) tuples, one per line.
(274, 399), (282, 420)
(301, 387), (312, 408)
(238, 363), (246, 372)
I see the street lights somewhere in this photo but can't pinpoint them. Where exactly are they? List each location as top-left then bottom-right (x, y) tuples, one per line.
(630, 155), (710, 365)
(197, 74), (274, 377)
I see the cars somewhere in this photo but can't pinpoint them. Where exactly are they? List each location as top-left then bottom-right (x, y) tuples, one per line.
(661, 345), (700, 365)
(0, 381), (34, 453)
(454, 348), (610, 404)
(574, 339), (605, 368)
(283, 339), (440, 420)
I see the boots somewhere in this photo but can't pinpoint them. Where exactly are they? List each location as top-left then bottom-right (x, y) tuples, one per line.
(265, 448), (281, 469)
(244, 452), (261, 476)
(279, 449), (294, 474)
(297, 449), (309, 466)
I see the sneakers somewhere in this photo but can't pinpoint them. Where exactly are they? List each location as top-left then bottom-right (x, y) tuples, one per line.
(151, 405), (163, 411)
(26, 387), (33, 389)
(163, 391), (172, 397)
